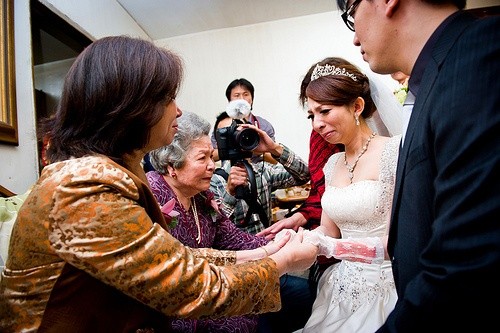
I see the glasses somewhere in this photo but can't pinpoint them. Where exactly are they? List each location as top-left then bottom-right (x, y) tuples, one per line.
(340, 0), (364, 32)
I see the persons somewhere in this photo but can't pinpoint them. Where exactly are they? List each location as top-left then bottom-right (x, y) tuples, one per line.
(210, 78), (274, 161)
(273, 55), (404, 333)
(336, 0), (500, 333)
(209, 111), (317, 333)
(256, 127), (344, 293)
(144, 108), (274, 333)
(0, 33), (321, 333)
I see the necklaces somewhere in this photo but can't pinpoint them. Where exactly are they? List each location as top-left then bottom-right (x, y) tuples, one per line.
(191, 195), (201, 243)
(344, 131), (377, 184)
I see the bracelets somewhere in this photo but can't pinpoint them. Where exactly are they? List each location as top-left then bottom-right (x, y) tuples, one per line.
(262, 246), (269, 256)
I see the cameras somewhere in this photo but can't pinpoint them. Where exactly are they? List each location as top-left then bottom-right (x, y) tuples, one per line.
(216, 126), (260, 160)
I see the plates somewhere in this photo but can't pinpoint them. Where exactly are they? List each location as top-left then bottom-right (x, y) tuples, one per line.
(275, 184), (311, 201)
